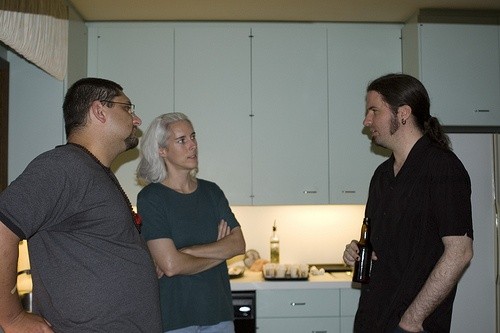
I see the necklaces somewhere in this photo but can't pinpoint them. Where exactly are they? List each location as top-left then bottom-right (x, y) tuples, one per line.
(71, 142), (143, 232)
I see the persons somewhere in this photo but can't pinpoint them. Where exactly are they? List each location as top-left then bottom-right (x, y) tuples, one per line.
(0, 77), (162, 333)
(134, 112), (246, 333)
(343, 73), (474, 333)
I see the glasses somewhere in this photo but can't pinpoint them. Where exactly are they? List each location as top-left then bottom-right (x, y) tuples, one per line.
(99, 99), (137, 117)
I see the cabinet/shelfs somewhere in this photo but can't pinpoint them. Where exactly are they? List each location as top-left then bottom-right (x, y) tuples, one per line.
(85, 22), (405, 205)
(229, 267), (361, 333)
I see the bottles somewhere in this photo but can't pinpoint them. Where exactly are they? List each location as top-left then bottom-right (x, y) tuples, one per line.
(271, 218), (279, 262)
(351, 217), (373, 291)
